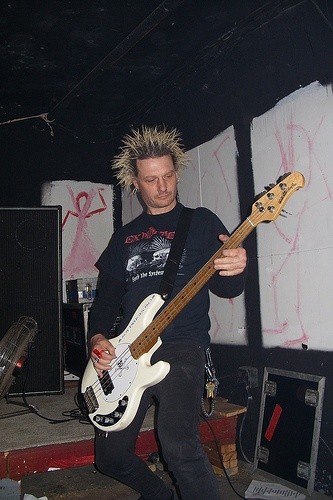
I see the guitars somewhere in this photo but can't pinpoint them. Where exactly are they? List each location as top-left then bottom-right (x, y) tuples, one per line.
(81, 172), (305, 433)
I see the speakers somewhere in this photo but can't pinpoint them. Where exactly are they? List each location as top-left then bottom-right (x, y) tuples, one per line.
(0, 205), (65, 397)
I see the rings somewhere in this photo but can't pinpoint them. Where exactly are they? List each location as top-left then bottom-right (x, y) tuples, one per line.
(95, 357), (100, 363)
(93, 360), (97, 366)
(93, 348), (105, 358)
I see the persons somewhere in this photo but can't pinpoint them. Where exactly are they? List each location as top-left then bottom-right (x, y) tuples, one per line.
(81, 128), (247, 500)
